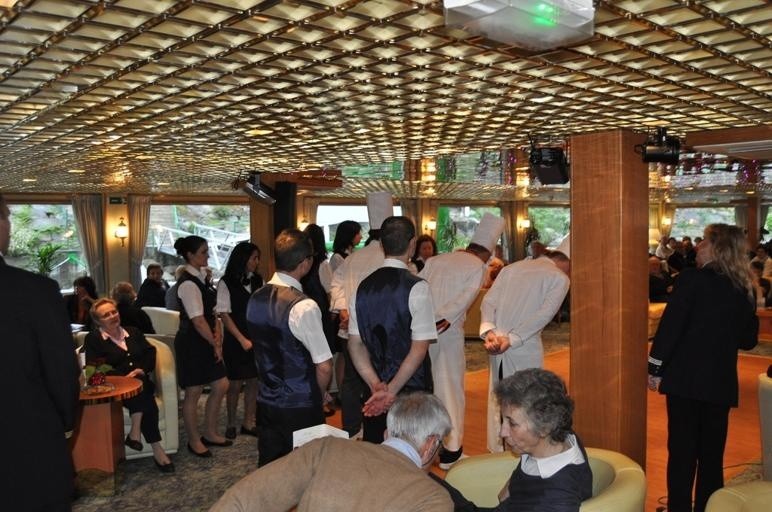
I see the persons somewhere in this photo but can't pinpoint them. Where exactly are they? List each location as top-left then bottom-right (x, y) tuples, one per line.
(647, 222), (759, 512)
(1, 196), (82, 512)
(207, 390), (457, 512)
(74, 215), (573, 474)
(652, 233), (772, 307)
(428, 369), (594, 511)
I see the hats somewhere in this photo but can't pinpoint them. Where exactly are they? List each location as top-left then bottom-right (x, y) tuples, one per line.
(364, 191), (393, 230)
(553, 231), (571, 260)
(469, 211), (506, 255)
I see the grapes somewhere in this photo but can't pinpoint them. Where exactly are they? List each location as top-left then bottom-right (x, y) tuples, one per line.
(87, 372), (104, 386)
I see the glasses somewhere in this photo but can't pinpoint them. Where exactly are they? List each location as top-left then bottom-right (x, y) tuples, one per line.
(436, 439), (444, 455)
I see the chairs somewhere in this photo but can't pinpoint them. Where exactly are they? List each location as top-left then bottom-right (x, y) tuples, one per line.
(443, 442), (651, 512)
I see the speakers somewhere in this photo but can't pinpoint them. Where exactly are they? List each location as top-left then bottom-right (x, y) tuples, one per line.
(530, 148), (569, 184)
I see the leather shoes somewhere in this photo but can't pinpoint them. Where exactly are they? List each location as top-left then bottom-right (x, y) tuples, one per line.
(188, 441), (213, 457)
(153, 458), (175, 472)
(239, 425), (258, 436)
(125, 433), (143, 451)
(200, 435), (233, 446)
(225, 424), (236, 439)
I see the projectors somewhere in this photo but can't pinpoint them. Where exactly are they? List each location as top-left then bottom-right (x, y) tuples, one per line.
(242, 171), (277, 206)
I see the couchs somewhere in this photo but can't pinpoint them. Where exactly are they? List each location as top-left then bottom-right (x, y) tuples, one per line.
(140, 306), (181, 336)
(704, 373), (772, 512)
(74, 331), (180, 461)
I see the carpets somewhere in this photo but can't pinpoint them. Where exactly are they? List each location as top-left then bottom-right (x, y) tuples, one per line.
(301, 343), (772, 512)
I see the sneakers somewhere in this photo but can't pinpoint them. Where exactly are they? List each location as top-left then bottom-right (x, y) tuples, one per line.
(439, 453), (470, 471)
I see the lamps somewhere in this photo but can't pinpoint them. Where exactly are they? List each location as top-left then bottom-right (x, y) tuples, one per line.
(114, 217), (129, 246)
(519, 215), (531, 229)
(441, 0), (598, 55)
(424, 215), (437, 231)
